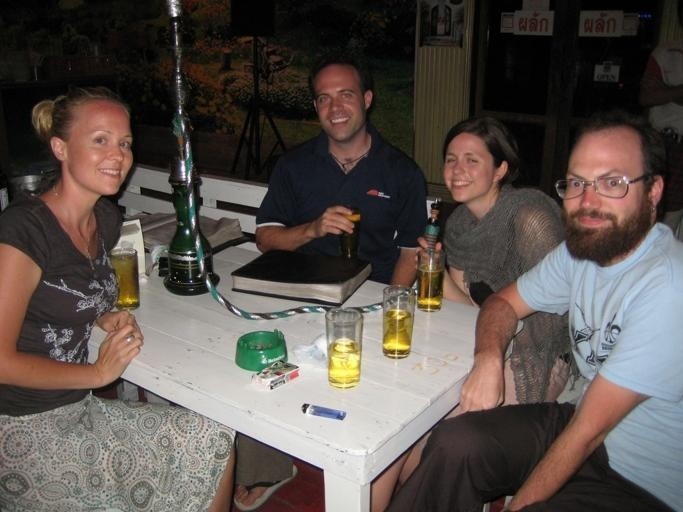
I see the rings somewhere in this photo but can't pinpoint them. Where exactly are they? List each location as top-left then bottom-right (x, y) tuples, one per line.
(125, 335), (134, 340)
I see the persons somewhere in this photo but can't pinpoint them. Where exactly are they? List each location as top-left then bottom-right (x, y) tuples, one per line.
(0, 87), (238, 512)
(417, 121), (567, 309)
(639, 1), (683, 242)
(385, 108), (683, 512)
(233, 52), (429, 511)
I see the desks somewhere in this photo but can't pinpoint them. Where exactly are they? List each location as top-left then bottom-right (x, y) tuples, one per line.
(82, 215), (524, 511)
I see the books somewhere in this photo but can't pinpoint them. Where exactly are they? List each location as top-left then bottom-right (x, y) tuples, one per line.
(230, 248), (373, 305)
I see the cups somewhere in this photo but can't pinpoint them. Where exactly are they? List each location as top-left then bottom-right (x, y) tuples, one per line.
(109, 248), (140, 311)
(417, 248), (444, 313)
(337, 206), (361, 259)
(381, 285), (418, 359)
(325, 309), (364, 387)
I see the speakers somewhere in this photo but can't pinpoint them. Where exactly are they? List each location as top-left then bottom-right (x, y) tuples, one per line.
(231, 0), (265, 37)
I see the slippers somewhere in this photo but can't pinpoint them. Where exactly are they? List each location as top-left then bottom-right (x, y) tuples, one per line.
(233, 463), (298, 510)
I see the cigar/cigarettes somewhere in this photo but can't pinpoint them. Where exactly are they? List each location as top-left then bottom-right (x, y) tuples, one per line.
(342, 214), (360, 221)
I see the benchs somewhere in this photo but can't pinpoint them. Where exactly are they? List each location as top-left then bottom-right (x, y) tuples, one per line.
(114, 162), (442, 288)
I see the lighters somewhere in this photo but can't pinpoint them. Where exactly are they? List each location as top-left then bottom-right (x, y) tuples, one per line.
(302, 403), (344, 421)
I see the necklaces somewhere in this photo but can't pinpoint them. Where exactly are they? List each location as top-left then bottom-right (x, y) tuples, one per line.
(52, 182), (96, 275)
(329, 150), (370, 171)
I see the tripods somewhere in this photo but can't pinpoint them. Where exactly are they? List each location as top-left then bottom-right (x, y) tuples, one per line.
(231, 46), (285, 179)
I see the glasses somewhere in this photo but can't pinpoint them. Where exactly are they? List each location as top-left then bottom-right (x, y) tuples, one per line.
(555, 174), (646, 200)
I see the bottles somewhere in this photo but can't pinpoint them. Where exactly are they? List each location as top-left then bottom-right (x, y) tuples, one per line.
(424, 199), (443, 252)
